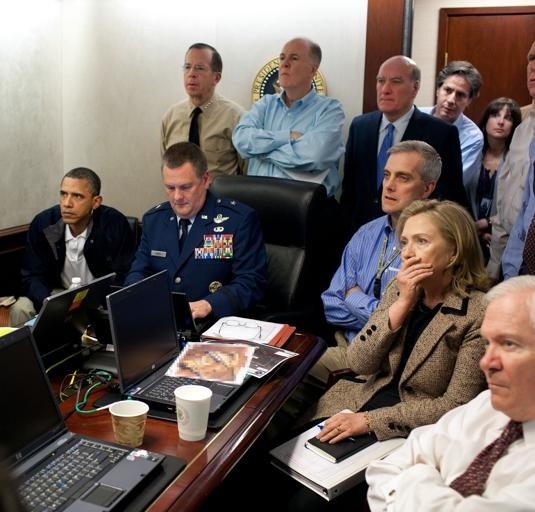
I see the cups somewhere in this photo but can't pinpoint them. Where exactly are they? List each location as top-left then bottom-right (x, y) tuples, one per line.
(109, 399), (150, 448)
(173, 384), (213, 441)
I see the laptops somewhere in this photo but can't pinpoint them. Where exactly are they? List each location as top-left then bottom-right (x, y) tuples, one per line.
(29, 271), (116, 363)
(107, 269), (252, 414)
(109, 285), (203, 340)
(0, 324), (167, 511)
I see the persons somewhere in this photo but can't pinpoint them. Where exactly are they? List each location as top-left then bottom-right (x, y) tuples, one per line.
(231, 38), (346, 279)
(495, 137), (535, 281)
(6, 164), (135, 329)
(261, 196), (497, 511)
(416, 62), (489, 207)
(482, 42), (535, 290)
(472, 96), (523, 263)
(262, 138), (446, 433)
(337, 54), (464, 250)
(156, 44), (251, 176)
(119, 142), (266, 327)
(361, 272), (534, 512)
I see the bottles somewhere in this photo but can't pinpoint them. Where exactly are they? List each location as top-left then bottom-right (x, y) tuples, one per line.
(69, 277), (83, 290)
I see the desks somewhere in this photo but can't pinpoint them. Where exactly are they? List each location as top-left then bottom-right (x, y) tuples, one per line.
(1, 305), (328, 511)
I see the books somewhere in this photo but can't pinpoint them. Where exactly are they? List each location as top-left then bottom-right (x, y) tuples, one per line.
(199, 313), (296, 349)
(304, 422), (377, 463)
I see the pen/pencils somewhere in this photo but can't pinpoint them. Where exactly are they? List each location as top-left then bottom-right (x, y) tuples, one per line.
(317, 425), (356, 442)
(388, 266), (400, 272)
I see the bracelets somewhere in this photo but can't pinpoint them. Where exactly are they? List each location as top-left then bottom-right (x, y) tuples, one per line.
(364, 409), (372, 437)
(487, 219), (493, 230)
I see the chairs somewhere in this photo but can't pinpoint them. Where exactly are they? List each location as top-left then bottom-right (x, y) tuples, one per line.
(208, 174), (328, 335)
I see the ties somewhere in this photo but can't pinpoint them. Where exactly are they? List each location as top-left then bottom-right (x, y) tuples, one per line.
(450, 418), (525, 497)
(188, 107), (202, 146)
(377, 124), (395, 189)
(178, 219), (191, 254)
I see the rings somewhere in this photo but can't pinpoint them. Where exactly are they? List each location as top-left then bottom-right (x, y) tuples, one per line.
(336, 425), (343, 432)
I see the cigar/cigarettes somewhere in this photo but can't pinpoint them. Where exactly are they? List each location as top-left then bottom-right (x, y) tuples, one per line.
(388, 266), (402, 273)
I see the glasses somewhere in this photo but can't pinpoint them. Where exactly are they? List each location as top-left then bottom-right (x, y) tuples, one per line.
(219, 320), (262, 341)
(181, 63), (217, 76)
